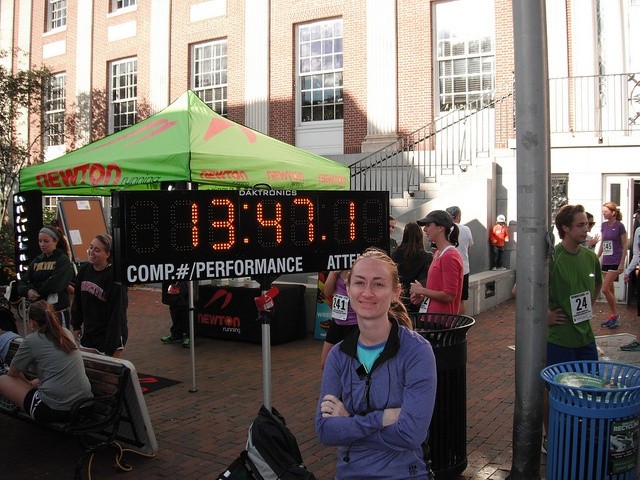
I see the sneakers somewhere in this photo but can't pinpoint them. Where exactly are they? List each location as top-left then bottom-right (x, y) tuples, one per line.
(601, 320), (619, 328)
(182, 335), (190, 347)
(492, 266), (496, 271)
(497, 266), (506, 271)
(619, 339), (640, 351)
(607, 315), (620, 328)
(160, 335), (183, 344)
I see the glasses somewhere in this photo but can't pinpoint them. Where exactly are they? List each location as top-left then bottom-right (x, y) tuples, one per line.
(589, 222), (595, 226)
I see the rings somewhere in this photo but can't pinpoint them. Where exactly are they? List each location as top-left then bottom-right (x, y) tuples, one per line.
(328, 407), (335, 415)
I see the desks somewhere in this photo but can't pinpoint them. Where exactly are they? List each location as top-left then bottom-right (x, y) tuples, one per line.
(192, 284), (308, 346)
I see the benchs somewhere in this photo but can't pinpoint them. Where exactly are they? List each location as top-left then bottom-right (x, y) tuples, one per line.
(0, 341), (131, 480)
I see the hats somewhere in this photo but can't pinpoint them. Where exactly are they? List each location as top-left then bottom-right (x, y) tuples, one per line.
(497, 214), (505, 223)
(416, 210), (453, 229)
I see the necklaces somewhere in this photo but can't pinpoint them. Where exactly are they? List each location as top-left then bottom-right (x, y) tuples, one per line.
(94, 263), (109, 270)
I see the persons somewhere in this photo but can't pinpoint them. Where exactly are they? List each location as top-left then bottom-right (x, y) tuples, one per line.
(388, 215), (398, 254)
(160, 278), (196, 348)
(444, 206), (474, 315)
(512, 204), (603, 456)
(17, 225), (72, 333)
(0, 300), (95, 425)
(320, 270), (358, 372)
(69, 233), (129, 357)
(409, 209), (464, 329)
(314, 250), (437, 478)
(581, 211), (600, 253)
(489, 214), (510, 271)
(619, 205), (639, 352)
(391, 222), (434, 329)
(595, 203), (628, 329)
(431, 240), (439, 256)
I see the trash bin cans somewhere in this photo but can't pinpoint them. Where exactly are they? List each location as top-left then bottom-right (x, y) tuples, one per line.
(408, 312), (475, 479)
(540, 361), (640, 480)
